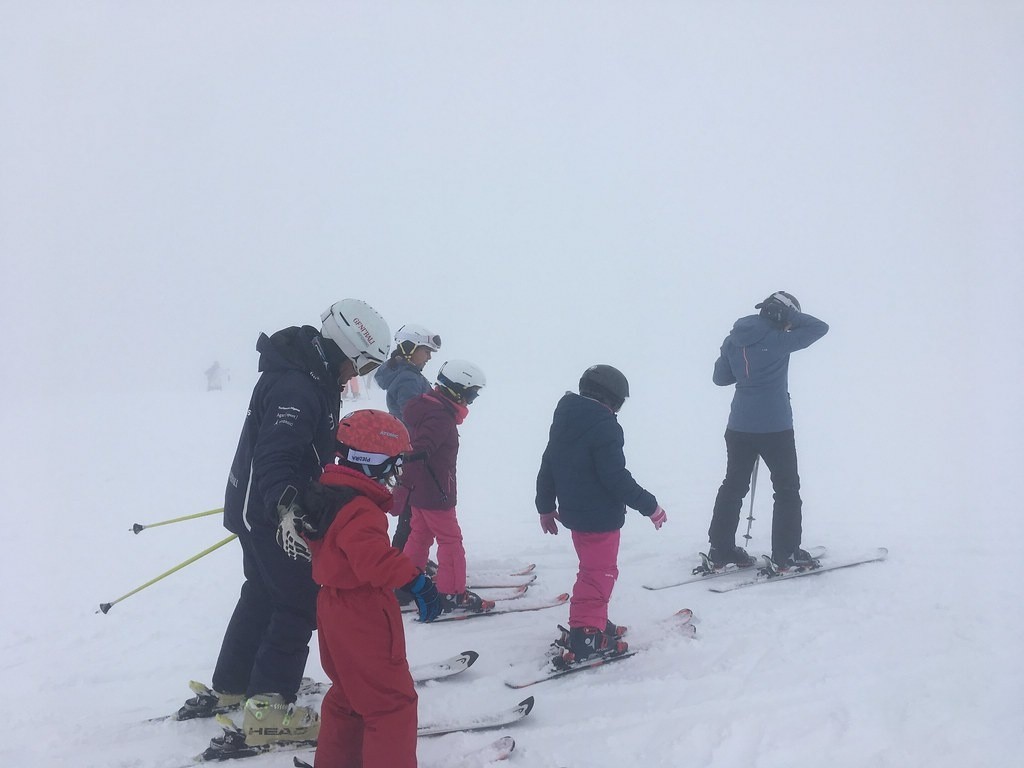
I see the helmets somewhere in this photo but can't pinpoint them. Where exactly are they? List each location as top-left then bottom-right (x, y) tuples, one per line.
(396, 323), (439, 352)
(436, 358), (485, 391)
(580, 364), (630, 412)
(321, 299), (390, 363)
(763, 292), (801, 327)
(335, 409), (413, 462)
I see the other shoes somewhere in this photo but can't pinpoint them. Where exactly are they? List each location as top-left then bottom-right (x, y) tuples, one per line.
(708, 545), (756, 568)
(772, 547), (811, 565)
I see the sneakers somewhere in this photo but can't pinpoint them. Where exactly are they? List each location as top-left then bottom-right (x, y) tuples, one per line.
(242, 695), (321, 743)
(604, 620), (626, 638)
(205, 687), (247, 710)
(437, 589), (496, 614)
(570, 627), (629, 656)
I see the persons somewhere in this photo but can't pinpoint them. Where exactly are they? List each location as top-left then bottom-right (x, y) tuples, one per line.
(535, 364), (667, 669)
(395, 358), (496, 612)
(375, 324), (442, 575)
(699, 291), (830, 576)
(293, 408), (446, 768)
(185, 296), (392, 750)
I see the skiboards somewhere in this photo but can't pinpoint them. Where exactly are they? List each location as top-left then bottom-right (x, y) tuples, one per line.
(504, 607), (697, 689)
(642, 546), (888, 593)
(161, 649), (536, 766)
(401, 564), (569, 626)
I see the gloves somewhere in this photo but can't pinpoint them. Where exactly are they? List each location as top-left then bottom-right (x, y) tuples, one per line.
(754, 300), (795, 323)
(404, 575), (442, 623)
(276, 484), (318, 563)
(540, 512), (562, 535)
(650, 505), (668, 530)
(389, 483), (410, 516)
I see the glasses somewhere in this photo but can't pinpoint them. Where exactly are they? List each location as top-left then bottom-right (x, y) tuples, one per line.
(464, 389), (478, 405)
(431, 334), (441, 349)
(357, 358), (382, 376)
